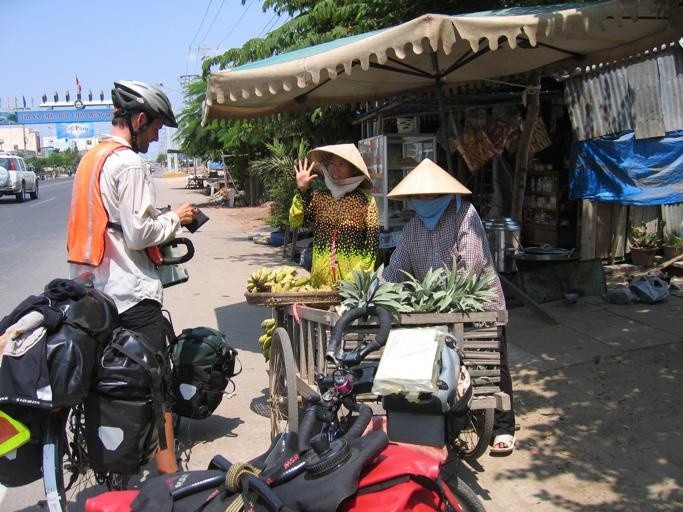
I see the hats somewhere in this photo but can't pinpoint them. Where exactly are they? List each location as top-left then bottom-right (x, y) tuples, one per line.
(385, 157), (474, 202)
(306, 141), (374, 190)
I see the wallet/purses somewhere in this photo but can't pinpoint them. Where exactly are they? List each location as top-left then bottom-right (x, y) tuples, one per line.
(180, 207), (210, 235)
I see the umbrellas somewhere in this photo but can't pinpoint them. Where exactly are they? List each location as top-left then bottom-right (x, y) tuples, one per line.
(199, 1), (683, 176)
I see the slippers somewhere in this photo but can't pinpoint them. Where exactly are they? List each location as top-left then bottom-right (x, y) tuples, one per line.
(490, 433), (516, 455)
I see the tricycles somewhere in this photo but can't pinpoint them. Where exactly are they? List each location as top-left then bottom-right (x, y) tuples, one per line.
(268, 302), (510, 460)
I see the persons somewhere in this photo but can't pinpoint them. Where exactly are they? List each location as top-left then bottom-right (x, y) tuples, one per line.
(374, 158), (519, 457)
(287, 142), (381, 292)
(65, 79), (198, 477)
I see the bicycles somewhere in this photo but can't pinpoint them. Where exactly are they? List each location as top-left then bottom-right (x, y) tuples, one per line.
(169, 304), (483, 511)
(42, 238), (193, 512)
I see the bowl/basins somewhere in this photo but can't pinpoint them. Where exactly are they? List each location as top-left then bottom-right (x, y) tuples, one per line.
(565, 294), (578, 301)
(396, 118), (414, 133)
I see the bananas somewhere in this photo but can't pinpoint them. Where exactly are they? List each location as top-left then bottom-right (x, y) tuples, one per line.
(247, 266), (315, 294)
(258, 319), (278, 363)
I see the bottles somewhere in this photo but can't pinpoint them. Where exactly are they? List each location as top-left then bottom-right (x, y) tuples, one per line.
(529, 176), (556, 192)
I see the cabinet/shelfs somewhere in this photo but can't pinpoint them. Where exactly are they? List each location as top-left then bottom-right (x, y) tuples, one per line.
(357, 133), (440, 234)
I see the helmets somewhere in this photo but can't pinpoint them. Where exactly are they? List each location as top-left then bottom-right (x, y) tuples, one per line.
(111, 78), (179, 128)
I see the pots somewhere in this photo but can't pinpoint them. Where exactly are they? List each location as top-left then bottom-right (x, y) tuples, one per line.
(524, 244), (570, 258)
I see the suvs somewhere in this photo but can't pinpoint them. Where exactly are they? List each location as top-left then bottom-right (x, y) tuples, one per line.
(0, 156), (38, 201)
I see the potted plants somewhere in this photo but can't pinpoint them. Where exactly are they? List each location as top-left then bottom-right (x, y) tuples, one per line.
(661, 226), (683, 263)
(624, 216), (667, 267)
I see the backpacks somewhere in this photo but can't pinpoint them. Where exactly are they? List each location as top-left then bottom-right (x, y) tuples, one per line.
(83, 329), (166, 475)
(168, 325), (236, 421)
(44, 274), (119, 414)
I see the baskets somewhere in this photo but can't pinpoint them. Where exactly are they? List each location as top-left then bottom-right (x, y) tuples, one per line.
(243, 288), (346, 305)
